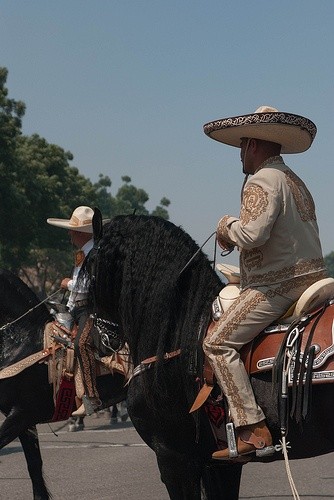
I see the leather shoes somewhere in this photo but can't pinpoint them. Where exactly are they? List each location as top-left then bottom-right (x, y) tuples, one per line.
(211, 419), (276, 461)
(72, 402), (89, 417)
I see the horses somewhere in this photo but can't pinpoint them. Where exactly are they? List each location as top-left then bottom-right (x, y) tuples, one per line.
(87, 206), (333, 500)
(0, 267), (127, 500)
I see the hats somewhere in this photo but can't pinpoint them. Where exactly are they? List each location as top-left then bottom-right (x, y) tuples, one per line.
(46, 204), (115, 235)
(204, 105), (317, 155)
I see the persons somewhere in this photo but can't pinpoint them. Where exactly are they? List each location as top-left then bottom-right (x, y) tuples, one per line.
(44, 206), (103, 419)
(201, 104), (328, 462)
